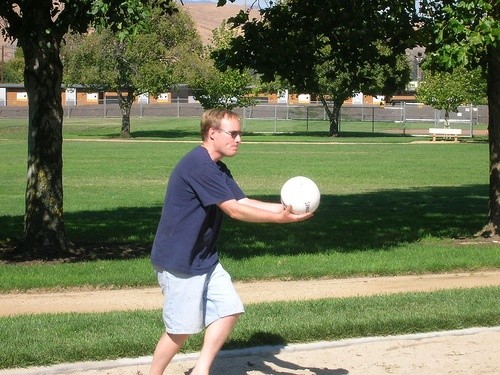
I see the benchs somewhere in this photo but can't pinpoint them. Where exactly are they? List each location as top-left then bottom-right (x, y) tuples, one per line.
(429, 128), (462, 142)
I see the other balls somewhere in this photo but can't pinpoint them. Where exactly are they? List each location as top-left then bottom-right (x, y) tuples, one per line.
(280, 175), (321, 215)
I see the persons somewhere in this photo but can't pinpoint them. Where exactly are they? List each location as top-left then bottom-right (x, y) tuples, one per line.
(149, 108), (314, 375)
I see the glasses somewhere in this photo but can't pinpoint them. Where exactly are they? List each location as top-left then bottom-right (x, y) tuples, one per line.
(207, 126), (242, 139)
